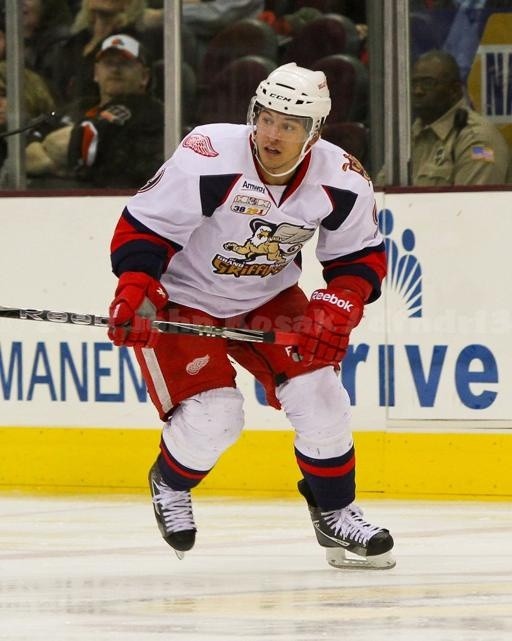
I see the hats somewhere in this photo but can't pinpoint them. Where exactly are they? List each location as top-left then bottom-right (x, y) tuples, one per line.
(96, 35), (150, 67)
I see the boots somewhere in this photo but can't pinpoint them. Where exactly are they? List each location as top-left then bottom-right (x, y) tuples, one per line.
(297, 479), (393, 557)
(149, 459), (197, 550)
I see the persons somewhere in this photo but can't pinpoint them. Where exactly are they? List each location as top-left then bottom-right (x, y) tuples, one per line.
(107, 62), (394, 557)
(0, 0), (512, 186)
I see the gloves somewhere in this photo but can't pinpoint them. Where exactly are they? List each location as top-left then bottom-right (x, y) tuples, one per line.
(108, 271), (169, 349)
(296, 289), (364, 369)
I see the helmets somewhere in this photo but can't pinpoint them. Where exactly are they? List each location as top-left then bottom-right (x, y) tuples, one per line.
(250, 62), (332, 157)
